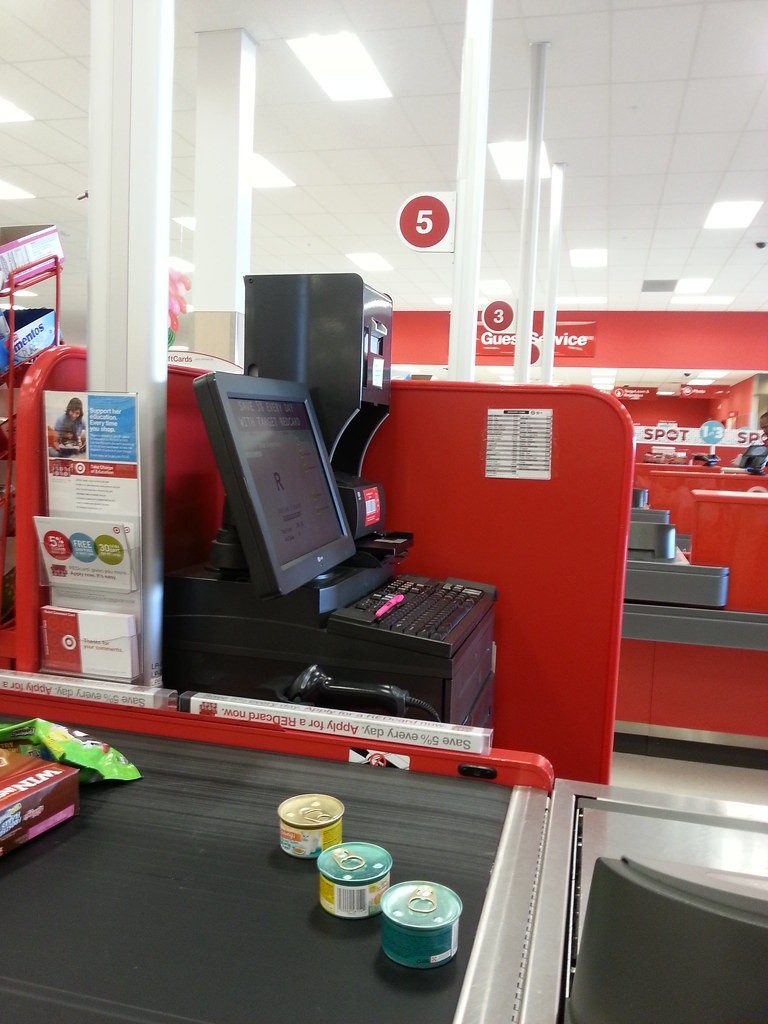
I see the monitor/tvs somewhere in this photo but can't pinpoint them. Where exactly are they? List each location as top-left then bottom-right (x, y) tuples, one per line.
(193, 370), (357, 601)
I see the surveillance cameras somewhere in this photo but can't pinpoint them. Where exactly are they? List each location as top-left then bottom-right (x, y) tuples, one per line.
(756, 242), (766, 249)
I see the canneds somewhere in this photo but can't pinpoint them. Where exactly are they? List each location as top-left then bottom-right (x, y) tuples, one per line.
(277, 794), (345, 859)
(380, 880), (464, 969)
(317, 841), (392, 917)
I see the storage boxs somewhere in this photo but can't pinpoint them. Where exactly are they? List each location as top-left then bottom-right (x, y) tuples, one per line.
(0, 748), (80, 857)
(0, 224), (65, 290)
(0, 310), (64, 375)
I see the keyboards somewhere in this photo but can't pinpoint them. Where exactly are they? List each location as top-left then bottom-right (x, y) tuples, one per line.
(325, 572), (495, 658)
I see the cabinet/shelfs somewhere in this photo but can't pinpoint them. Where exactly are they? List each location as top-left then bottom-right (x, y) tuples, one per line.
(0, 256), (64, 669)
